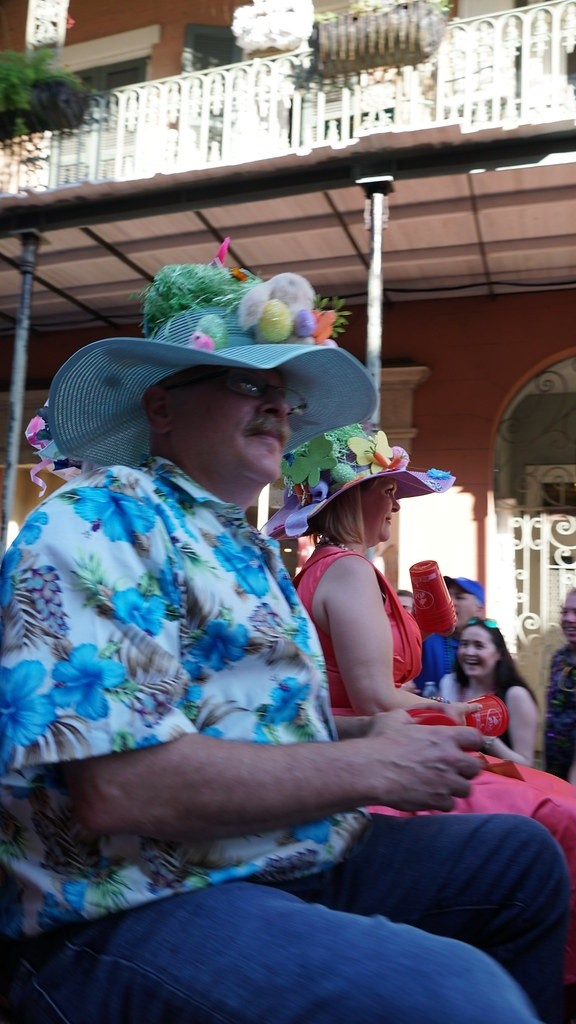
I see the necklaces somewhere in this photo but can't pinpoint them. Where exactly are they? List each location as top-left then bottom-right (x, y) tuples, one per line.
(325, 537), (349, 551)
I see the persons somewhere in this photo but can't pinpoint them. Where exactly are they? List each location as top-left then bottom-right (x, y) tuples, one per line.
(545, 586), (576, 786)
(395, 575), (539, 765)
(0, 264), (576, 1023)
(259, 423), (576, 989)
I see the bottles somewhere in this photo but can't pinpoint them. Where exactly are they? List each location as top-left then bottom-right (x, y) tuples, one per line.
(423, 681), (438, 698)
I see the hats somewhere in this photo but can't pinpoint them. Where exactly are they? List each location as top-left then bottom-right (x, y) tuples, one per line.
(444, 576), (483, 603)
(261, 423), (457, 539)
(47, 263), (379, 468)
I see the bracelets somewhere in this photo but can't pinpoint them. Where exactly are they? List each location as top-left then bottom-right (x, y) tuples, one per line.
(429, 696), (451, 705)
(485, 737), (494, 749)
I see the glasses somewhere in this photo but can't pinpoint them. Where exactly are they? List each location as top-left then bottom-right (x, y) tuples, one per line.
(171, 371), (308, 415)
(467, 616), (498, 629)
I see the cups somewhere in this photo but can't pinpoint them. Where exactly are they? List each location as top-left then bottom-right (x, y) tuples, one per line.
(407, 710), (457, 726)
(410, 560), (456, 633)
(464, 695), (508, 736)
